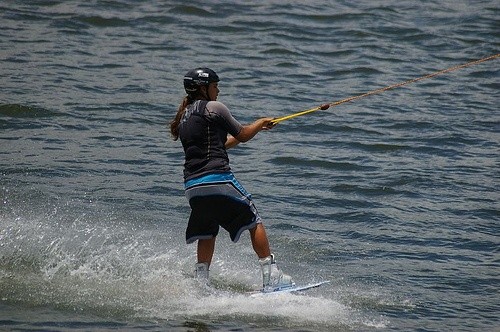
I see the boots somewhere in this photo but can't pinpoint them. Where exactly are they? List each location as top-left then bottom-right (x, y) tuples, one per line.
(259, 255), (296, 293)
(193, 262), (220, 300)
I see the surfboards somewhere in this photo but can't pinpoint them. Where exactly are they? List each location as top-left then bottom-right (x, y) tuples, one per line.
(249, 280), (329, 298)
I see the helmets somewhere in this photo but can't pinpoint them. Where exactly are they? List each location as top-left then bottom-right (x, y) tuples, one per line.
(183, 67), (221, 95)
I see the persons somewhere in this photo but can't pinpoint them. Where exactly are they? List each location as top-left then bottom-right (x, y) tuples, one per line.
(167, 66), (298, 293)
